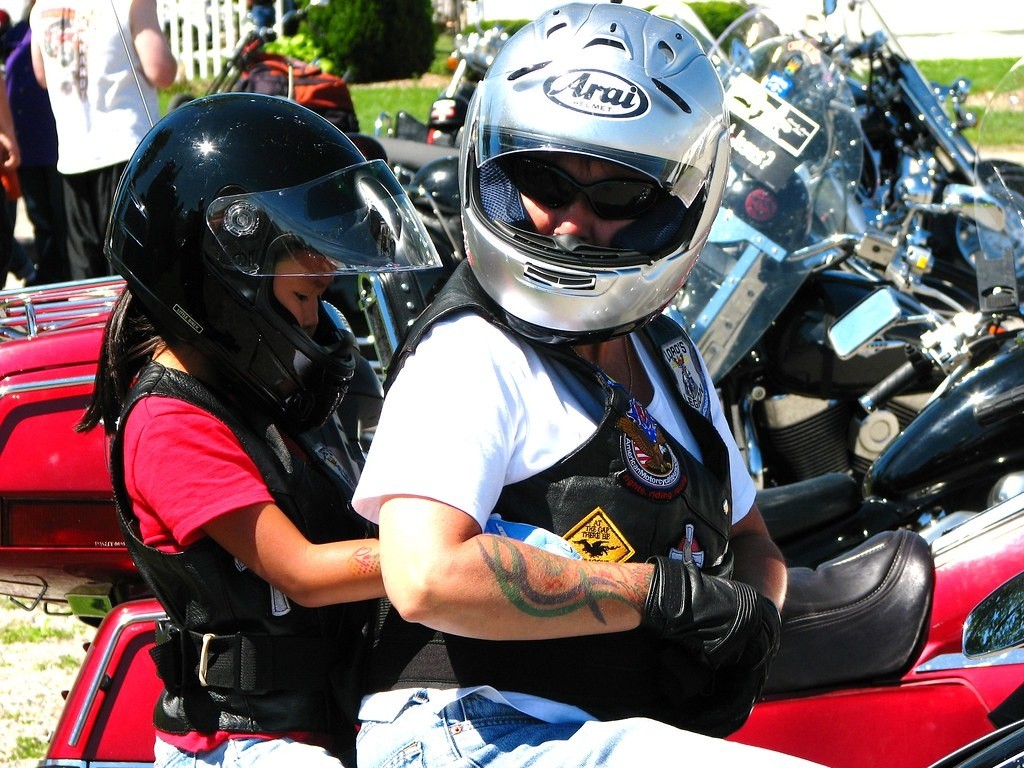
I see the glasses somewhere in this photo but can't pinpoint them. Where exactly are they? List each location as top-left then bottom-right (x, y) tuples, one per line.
(495, 154), (679, 224)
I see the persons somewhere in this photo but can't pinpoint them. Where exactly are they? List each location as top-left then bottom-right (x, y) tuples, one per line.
(351, 4), (830, 767)
(0, 0), (177, 290)
(75, 94), (580, 768)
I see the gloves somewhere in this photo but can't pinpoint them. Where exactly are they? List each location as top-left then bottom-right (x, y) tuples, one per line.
(640, 554), (783, 670)
(667, 658), (769, 740)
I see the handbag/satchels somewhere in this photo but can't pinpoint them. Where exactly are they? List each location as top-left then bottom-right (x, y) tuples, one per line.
(239, 67), (362, 132)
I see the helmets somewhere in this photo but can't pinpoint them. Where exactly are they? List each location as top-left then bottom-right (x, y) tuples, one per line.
(99, 92), (445, 442)
(457, 2), (731, 347)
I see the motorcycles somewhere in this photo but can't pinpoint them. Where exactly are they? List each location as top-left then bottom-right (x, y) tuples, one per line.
(0, 1), (1024, 767)
